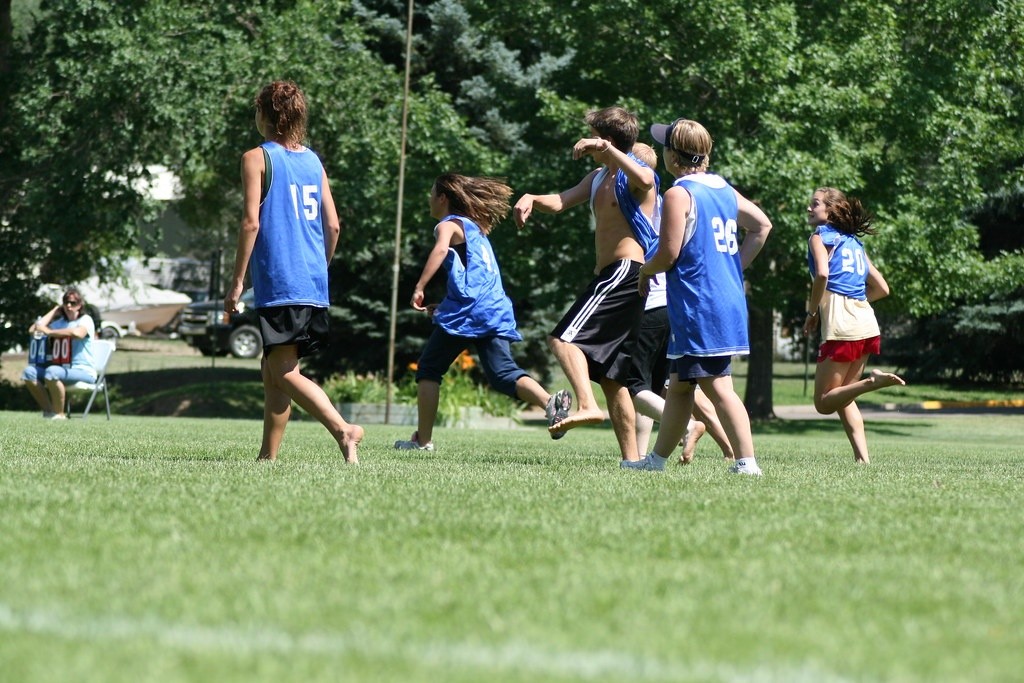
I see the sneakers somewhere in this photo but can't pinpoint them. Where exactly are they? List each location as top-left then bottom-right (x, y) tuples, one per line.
(394, 431), (434, 451)
(545, 390), (572, 440)
(728, 464), (762, 475)
(619, 457), (665, 472)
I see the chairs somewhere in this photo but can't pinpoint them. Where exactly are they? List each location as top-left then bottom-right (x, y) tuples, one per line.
(44, 338), (115, 421)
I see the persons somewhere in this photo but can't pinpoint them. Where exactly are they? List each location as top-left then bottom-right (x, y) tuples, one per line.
(394, 173), (573, 453)
(20, 287), (98, 422)
(220, 81), (365, 466)
(513, 106), (774, 477)
(800, 186), (908, 466)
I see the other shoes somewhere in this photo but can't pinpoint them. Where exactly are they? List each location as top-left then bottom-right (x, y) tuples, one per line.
(43, 410), (67, 420)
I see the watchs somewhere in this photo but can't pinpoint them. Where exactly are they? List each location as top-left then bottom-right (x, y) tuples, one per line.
(639, 265), (654, 278)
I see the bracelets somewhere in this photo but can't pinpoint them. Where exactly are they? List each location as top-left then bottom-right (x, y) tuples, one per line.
(808, 310), (819, 318)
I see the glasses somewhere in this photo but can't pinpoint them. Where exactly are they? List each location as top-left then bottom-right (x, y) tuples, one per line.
(63, 300), (79, 306)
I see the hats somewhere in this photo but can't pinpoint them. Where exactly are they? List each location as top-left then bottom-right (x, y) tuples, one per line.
(650, 117), (706, 165)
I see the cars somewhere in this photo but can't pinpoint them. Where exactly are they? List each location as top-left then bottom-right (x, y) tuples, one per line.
(177, 285), (263, 360)
